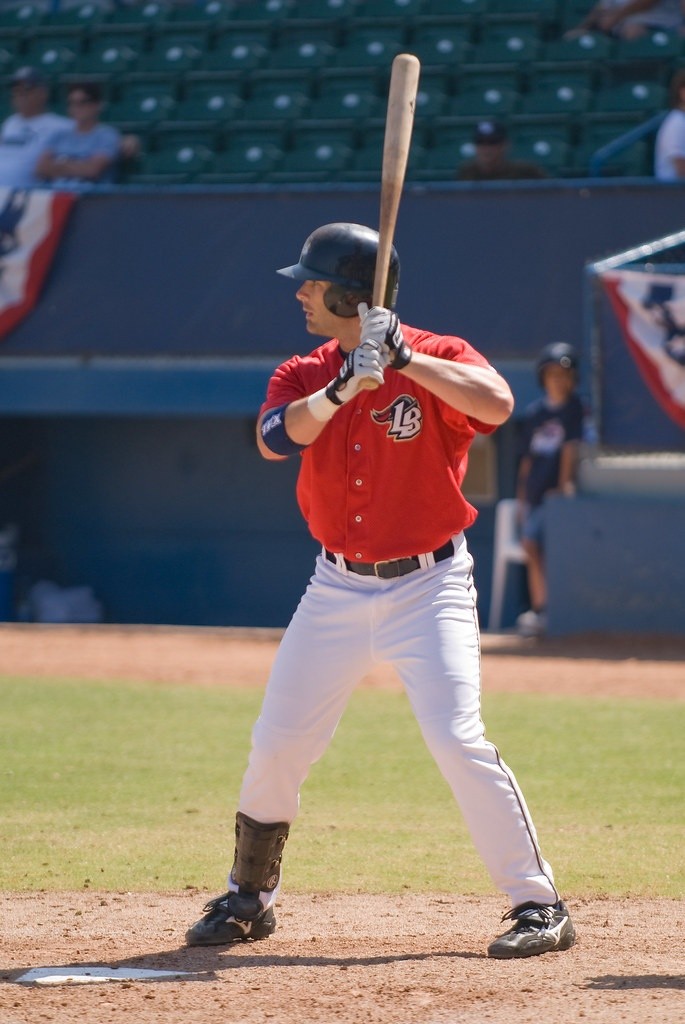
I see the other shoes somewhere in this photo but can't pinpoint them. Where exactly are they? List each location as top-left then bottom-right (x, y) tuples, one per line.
(519, 609), (545, 638)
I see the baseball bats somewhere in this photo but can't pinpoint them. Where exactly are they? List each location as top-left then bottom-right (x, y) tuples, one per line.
(359, 52), (424, 391)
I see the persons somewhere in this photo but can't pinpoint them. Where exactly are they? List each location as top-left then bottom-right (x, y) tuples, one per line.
(655, 69), (685, 190)
(562, 0), (685, 38)
(185, 222), (576, 959)
(13, 557), (109, 624)
(512, 343), (601, 639)
(452, 120), (549, 179)
(0, 66), (142, 195)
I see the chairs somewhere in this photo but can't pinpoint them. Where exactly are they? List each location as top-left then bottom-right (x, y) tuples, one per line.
(1, 0), (684, 182)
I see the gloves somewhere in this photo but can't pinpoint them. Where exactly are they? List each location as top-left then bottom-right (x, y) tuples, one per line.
(325, 339), (391, 407)
(357, 302), (412, 370)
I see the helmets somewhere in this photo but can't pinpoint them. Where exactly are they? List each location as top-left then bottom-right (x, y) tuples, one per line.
(276, 222), (401, 318)
(536, 343), (579, 386)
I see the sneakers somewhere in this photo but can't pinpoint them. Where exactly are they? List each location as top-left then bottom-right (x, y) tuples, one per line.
(487, 899), (576, 959)
(185, 889), (276, 946)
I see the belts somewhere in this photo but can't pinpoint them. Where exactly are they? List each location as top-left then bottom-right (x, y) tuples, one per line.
(325, 540), (454, 581)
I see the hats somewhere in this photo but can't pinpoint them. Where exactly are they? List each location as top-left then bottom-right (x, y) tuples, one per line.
(8, 65), (58, 88)
